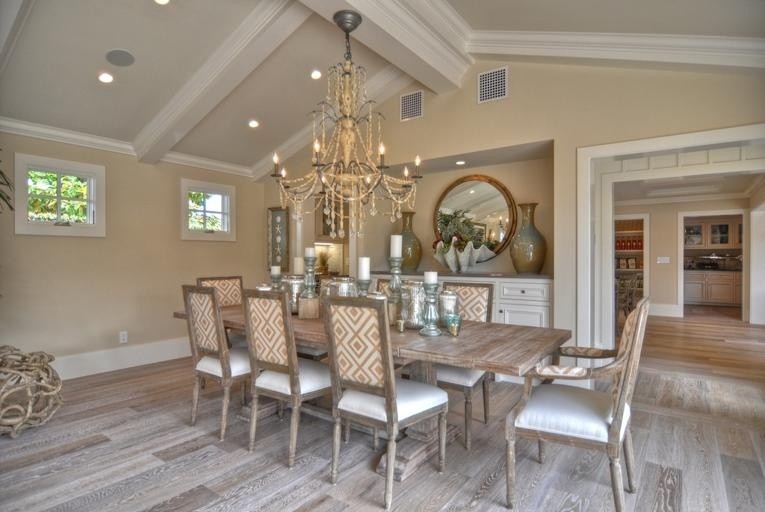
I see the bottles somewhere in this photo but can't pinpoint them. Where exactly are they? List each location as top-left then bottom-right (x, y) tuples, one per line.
(615, 236), (643, 250)
(689, 226), (694, 235)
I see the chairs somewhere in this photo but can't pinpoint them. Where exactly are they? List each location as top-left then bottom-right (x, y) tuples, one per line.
(321, 295), (451, 511)
(195, 275), (248, 388)
(181, 282), (285, 441)
(239, 287), (349, 469)
(504, 295), (652, 512)
(400, 282), (494, 451)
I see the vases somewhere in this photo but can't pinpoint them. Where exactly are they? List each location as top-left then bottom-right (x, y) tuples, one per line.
(509, 201), (545, 275)
(396, 211), (423, 275)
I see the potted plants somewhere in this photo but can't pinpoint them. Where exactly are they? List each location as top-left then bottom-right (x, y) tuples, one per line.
(431, 210), (497, 273)
(319, 252), (331, 276)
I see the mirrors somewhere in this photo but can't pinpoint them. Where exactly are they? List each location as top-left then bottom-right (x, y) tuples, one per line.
(432, 172), (517, 264)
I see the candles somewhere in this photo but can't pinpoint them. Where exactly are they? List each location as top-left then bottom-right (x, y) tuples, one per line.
(389, 234), (402, 258)
(424, 271), (438, 287)
(358, 256), (372, 281)
(304, 247), (314, 257)
(271, 265), (281, 275)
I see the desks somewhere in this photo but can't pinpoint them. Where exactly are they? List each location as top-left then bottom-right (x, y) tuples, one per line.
(173, 302), (572, 481)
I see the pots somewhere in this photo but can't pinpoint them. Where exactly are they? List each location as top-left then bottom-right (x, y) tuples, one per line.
(700, 263), (718, 269)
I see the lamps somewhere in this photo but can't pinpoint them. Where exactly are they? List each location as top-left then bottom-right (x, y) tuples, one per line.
(270, 9), (423, 238)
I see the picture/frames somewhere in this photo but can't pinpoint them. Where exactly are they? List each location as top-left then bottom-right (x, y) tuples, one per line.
(267, 206), (290, 273)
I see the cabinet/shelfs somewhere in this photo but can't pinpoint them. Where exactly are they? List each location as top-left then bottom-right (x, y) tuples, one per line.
(684, 271), (707, 305)
(684, 216), (733, 249)
(733, 216), (743, 249)
(370, 270), (424, 283)
(734, 272), (742, 307)
(436, 276), (554, 388)
(613, 213), (651, 300)
(707, 271), (734, 307)
